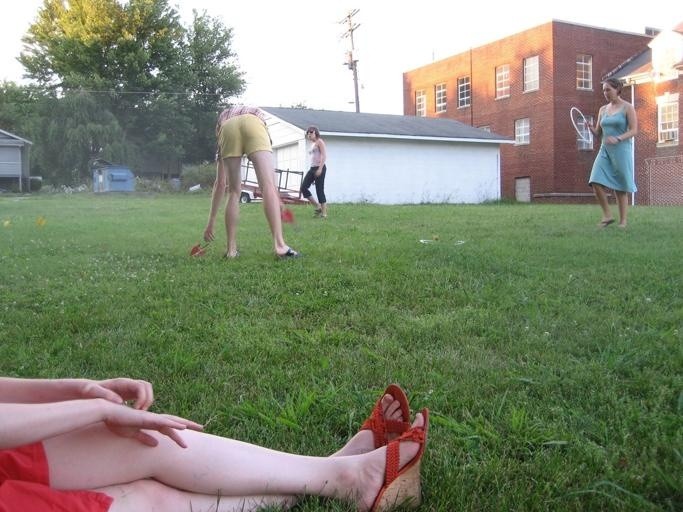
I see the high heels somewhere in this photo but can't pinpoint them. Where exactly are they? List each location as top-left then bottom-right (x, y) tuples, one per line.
(370, 406), (428, 511)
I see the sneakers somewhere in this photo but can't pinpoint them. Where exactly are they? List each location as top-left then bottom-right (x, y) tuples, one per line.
(312, 208), (327, 218)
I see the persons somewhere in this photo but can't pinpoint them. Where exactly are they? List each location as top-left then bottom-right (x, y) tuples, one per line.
(204, 105), (304, 259)
(300, 125), (327, 218)
(584, 77), (637, 228)
(0, 376), (428, 512)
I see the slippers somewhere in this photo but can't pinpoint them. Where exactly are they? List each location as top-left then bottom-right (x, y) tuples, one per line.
(275, 245), (298, 258)
(359, 383), (409, 449)
(222, 250), (240, 259)
(596, 217), (617, 231)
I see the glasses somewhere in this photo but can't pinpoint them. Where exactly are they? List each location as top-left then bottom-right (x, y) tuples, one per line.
(306, 131), (313, 135)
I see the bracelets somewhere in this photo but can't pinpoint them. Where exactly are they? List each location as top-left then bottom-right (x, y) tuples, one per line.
(615, 136), (622, 142)
